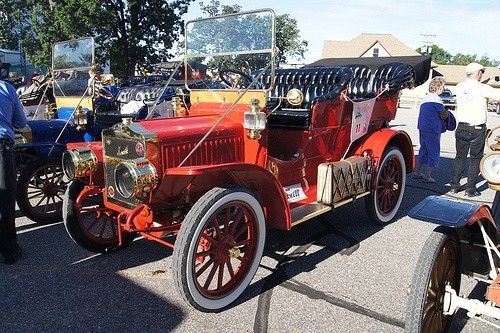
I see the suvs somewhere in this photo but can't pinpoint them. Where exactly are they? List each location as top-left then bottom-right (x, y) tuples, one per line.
(439, 89), (457, 111)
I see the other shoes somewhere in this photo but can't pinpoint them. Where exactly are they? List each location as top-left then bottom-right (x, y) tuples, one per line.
(464, 191), (482, 197)
(451, 187), (461, 194)
(6, 240), (22, 265)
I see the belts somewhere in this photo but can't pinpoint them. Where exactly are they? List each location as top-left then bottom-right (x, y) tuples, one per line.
(458, 121), (486, 130)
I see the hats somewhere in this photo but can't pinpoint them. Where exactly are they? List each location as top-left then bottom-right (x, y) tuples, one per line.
(465, 63), (486, 75)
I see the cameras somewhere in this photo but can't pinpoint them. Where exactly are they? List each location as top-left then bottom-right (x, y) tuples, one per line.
(495, 76), (500, 81)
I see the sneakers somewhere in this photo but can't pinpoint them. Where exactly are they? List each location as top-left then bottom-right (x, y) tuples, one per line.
(412, 172), (435, 183)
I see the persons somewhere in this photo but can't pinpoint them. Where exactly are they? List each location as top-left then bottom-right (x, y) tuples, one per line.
(0, 58), (70, 267)
(415, 74), (450, 181)
(449, 61), (500, 196)
(87, 64), (113, 96)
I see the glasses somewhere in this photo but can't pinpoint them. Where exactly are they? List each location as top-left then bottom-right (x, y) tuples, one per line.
(480, 69), (485, 74)
